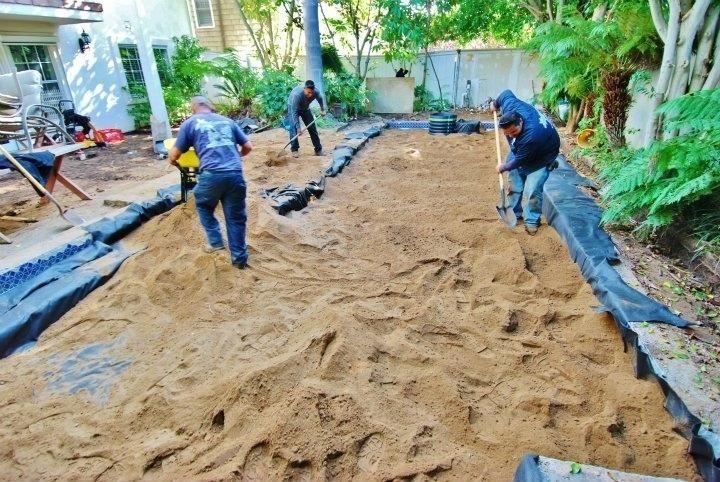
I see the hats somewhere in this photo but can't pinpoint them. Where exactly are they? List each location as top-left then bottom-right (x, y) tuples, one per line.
(304, 80), (315, 88)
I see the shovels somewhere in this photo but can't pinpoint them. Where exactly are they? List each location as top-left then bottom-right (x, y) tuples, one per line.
(0, 144), (86, 226)
(494, 111), (517, 229)
(268, 114), (322, 161)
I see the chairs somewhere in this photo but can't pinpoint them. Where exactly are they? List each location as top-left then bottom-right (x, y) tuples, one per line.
(0, 70), (58, 146)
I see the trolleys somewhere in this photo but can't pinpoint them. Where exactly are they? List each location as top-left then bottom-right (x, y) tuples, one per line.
(162, 136), (203, 203)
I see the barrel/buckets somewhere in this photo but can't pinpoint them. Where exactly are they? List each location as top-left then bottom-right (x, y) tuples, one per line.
(577, 128), (596, 149)
(429, 110), (458, 136)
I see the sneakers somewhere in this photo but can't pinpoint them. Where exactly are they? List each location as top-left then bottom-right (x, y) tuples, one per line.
(314, 149), (326, 156)
(290, 150), (298, 158)
(203, 246), (225, 253)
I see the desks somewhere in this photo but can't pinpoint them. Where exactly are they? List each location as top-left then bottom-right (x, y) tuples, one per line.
(0, 143), (93, 244)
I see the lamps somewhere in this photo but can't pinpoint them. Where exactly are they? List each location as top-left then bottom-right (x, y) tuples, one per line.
(79, 30), (91, 53)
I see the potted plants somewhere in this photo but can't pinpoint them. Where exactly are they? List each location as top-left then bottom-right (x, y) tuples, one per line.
(323, 69), (360, 117)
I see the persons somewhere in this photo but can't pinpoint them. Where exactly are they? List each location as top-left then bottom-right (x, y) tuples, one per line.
(169, 96), (252, 269)
(490, 89), (560, 228)
(287, 79), (326, 157)
